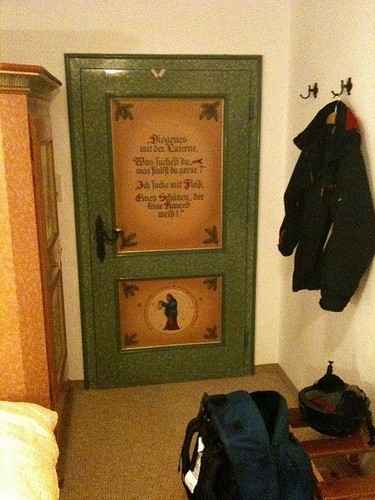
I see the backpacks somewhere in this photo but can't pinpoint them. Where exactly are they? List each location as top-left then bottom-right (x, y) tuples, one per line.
(177, 390), (325, 500)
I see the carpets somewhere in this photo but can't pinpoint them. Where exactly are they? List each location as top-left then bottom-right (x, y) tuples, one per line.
(287, 407), (375, 500)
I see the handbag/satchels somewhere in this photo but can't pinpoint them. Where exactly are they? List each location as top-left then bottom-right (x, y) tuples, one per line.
(297, 372), (375, 447)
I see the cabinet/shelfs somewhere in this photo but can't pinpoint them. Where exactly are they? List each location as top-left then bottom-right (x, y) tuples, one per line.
(0, 62), (73, 441)
